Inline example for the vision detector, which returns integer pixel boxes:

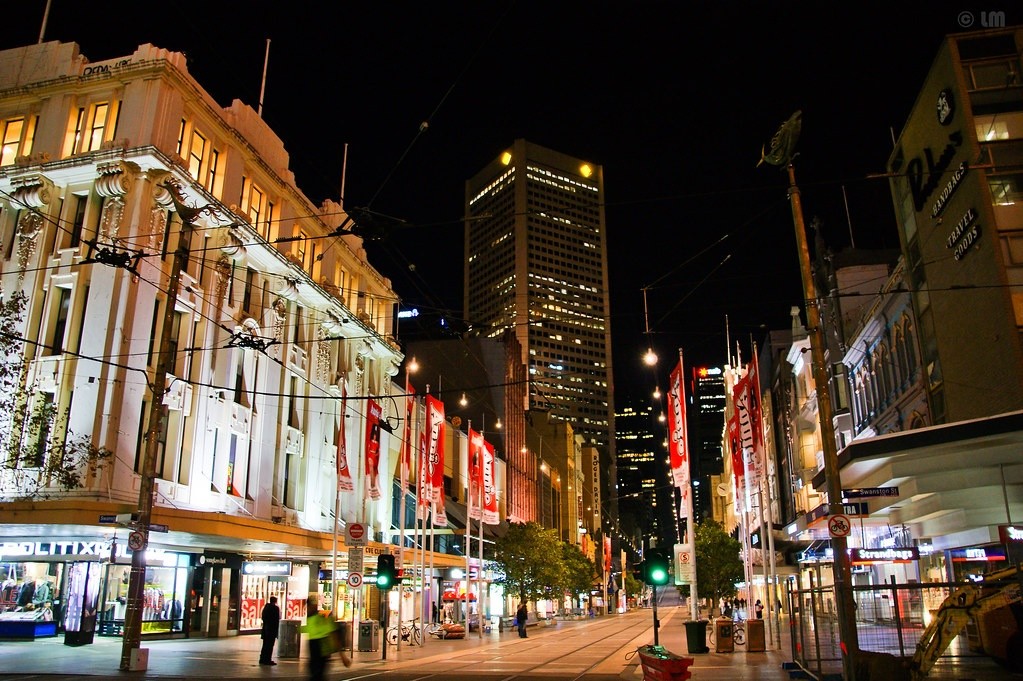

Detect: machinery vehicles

[852,562,1023,681]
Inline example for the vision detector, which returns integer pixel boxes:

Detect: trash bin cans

[713,616,734,652]
[683,621,711,653]
[358,620,379,653]
[278,619,301,659]
[334,620,352,652]
[744,619,766,651]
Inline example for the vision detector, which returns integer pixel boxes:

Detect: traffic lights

[646,547,669,586]
[376,554,395,590]
[632,559,647,582]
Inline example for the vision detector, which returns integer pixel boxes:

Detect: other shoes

[259,660,277,665]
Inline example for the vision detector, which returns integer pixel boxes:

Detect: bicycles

[386,616,425,645]
[709,617,745,645]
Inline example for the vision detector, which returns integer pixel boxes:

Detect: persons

[1,576,48,608]
[719,597,782,620]
[431,601,437,626]
[520,600,528,638]
[297,598,351,681]
[438,605,446,624]
[517,604,522,636]
[141,588,180,631]
[258,596,279,665]
